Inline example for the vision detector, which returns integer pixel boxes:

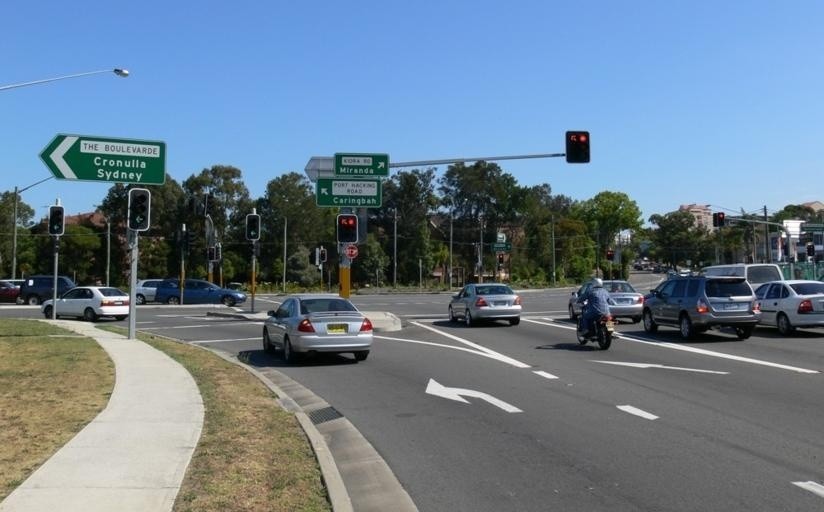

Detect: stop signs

[346,245,358,258]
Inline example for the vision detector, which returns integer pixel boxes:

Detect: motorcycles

[576,299,614,350]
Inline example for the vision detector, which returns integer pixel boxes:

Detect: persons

[578,278,610,336]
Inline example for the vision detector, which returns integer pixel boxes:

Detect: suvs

[643,275,762,338]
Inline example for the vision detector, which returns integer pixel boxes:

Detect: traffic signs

[491,243,511,252]
[334,153,389,177]
[315,178,382,208]
[38,133,165,185]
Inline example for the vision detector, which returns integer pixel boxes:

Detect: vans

[699,264,785,293]
[20,276,76,305]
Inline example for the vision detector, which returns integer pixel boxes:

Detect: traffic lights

[209,244,221,262]
[127,187,151,232]
[713,213,724,227]
[47,206,65,237]
[246,214,260,241]
[499,254,502,263]
[337,214,358,244]
[807,245,814,256]
[566,131,590,163]
[320,249,327,262]
[783,245,788,256]
[176,223,193,247]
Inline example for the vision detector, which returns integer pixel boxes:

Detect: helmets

[591,278,604,288]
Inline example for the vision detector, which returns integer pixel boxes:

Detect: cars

[755,279,824,336]
[136,279,247,306]
[568,280,644,323]
[633,262,692,278]
[40,286,130,323]
[262,295,373,364]
[0,281,24,305]
[448,284,522,327]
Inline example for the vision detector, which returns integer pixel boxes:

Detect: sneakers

[580,329,590,336]
